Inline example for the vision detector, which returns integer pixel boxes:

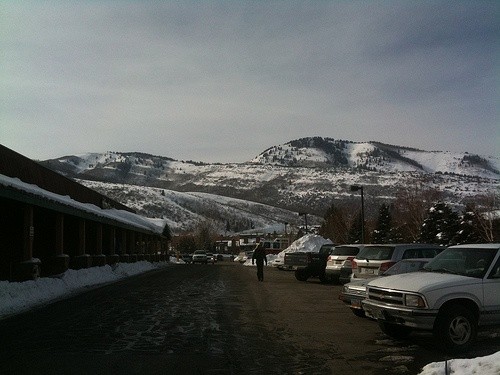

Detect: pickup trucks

[284,244,342,283]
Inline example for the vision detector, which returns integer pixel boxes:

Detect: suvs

[192,249,207,265]
[355,242,475,284]
[326,243,367,277]
[206,253,215,264]
[361,243,500,355]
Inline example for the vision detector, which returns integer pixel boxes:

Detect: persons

[251,242,268,282]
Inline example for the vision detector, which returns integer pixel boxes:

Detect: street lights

[351,184,365,244]
[300,210,309,236]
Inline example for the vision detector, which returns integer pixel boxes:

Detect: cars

[214,255,218,262]
[339,258,436,320]
[272,258,298,271]
